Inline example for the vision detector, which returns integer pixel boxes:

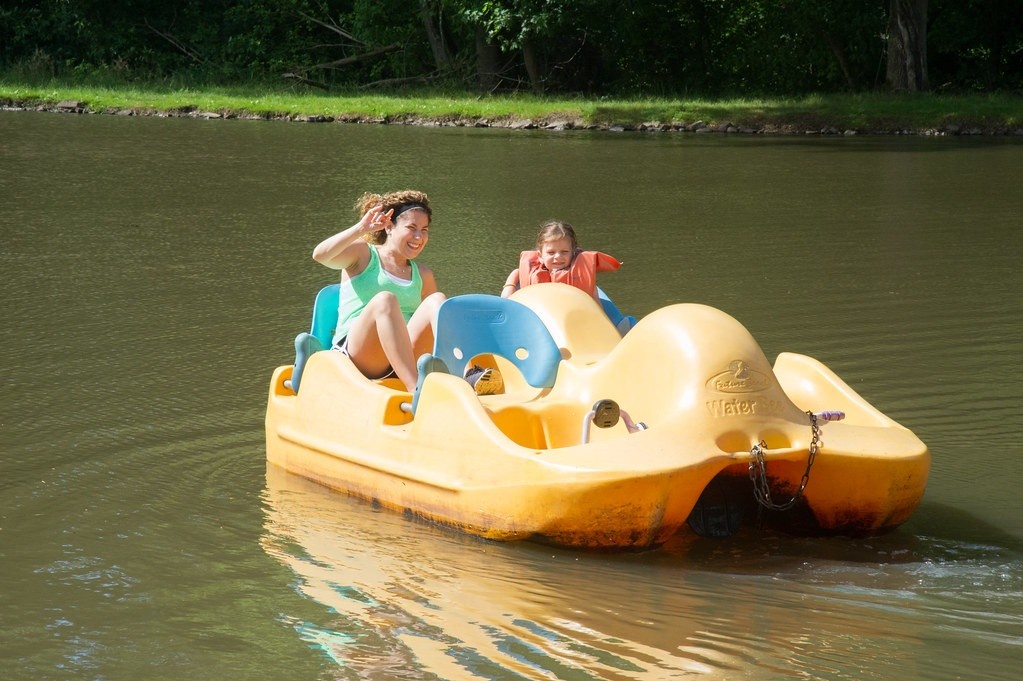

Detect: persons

[500,220,638,337]
[312,190,450,392]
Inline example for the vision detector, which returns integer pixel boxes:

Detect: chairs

[410,293,561,439]
[293,283,407,396]
[514,281,635,338]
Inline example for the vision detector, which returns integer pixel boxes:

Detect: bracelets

[502,284,517,288]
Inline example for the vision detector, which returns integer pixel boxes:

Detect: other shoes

[464,364,503,395]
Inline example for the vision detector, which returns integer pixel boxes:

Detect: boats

[264,283,933,549]
